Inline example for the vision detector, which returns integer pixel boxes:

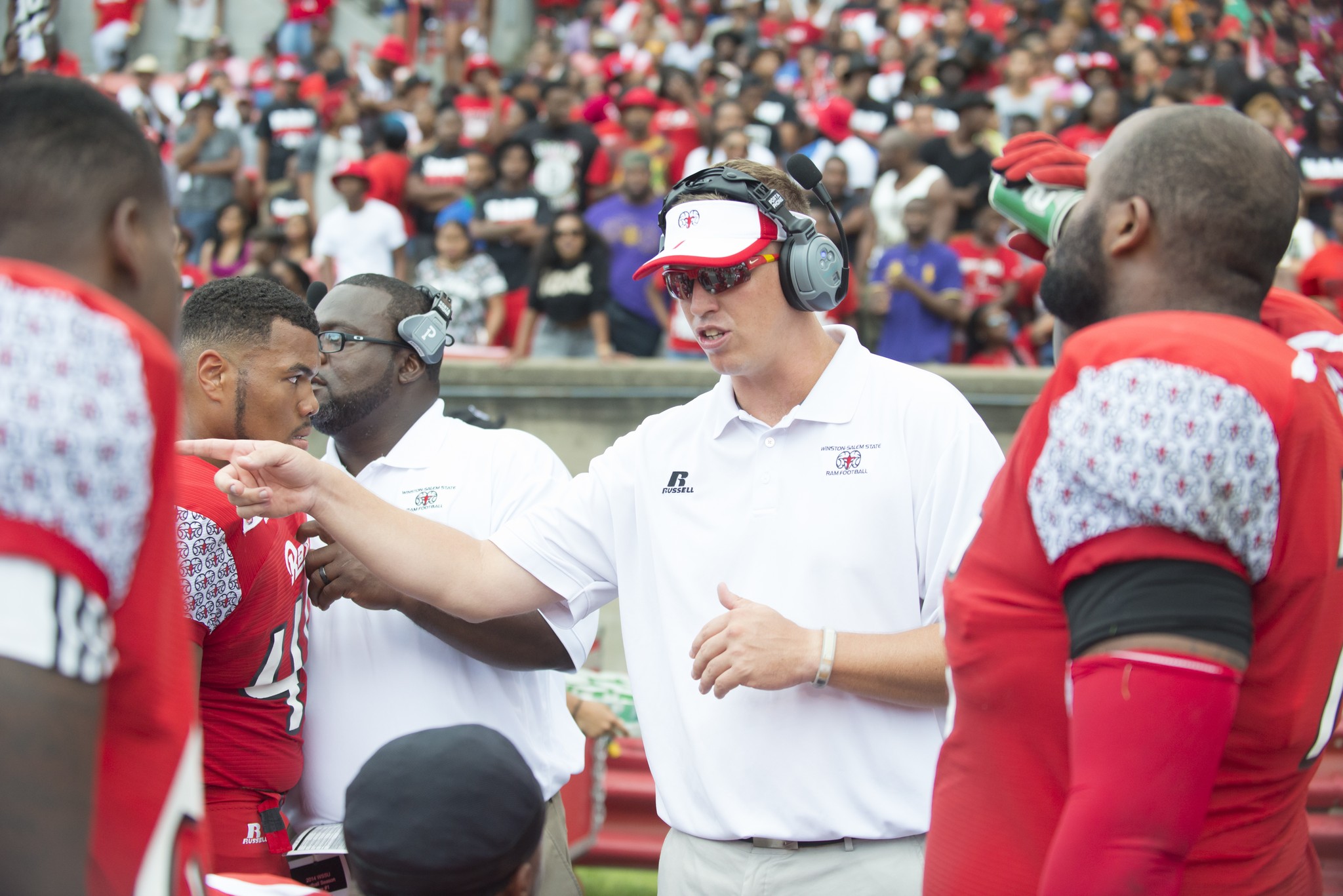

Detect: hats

[341,724,549,896]
[632,198,820,285]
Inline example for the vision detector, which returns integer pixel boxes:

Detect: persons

[285,272,600,895]
[0,0,1342,372]
[173,270,323,896]
[2,69,201,896]
[922,100,1343,896]
[340,722,553,896]
[562,690,631,743]
[171,160,1008,895]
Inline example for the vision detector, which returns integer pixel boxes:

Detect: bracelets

[571,695,584,722]
[811,627,838,690]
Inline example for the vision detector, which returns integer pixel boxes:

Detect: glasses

[661,254,779,299]
[317,330,416,354]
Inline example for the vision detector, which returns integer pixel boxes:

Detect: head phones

[397,284,453,364]
[657,167,848,311]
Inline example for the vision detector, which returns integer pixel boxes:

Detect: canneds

[987,166,1091,249]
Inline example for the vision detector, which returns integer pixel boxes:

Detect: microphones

[786,154,848,302]
[306,280,328,309]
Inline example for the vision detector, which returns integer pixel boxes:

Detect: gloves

[991,130,1093,264]
